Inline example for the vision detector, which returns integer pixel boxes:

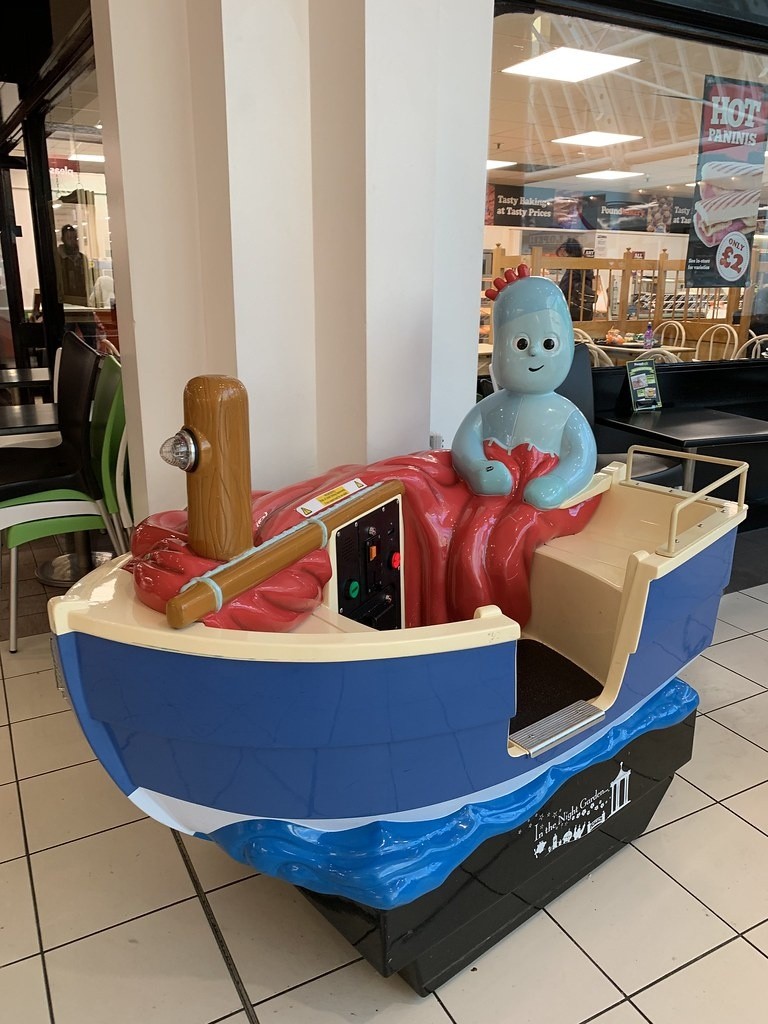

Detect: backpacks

[570,282,597,321]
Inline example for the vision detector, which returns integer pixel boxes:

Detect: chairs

[554,343,684,491]
[0,330,133,653]
[692,324,768,362]
[653,321,686,362]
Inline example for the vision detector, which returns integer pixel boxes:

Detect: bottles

[644,322,653,349]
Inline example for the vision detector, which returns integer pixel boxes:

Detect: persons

[55,225,98,348]
[551,237,596,323]
[751,273,768,356]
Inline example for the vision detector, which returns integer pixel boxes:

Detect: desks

[0,403,63,448]
[0,367,51,382]
[595,405,768,491]
[598,343,696,353]
[478,343,494,371]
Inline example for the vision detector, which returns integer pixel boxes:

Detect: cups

[608,329,619,343]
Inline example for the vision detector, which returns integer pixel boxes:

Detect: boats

[46,373,754,911]
